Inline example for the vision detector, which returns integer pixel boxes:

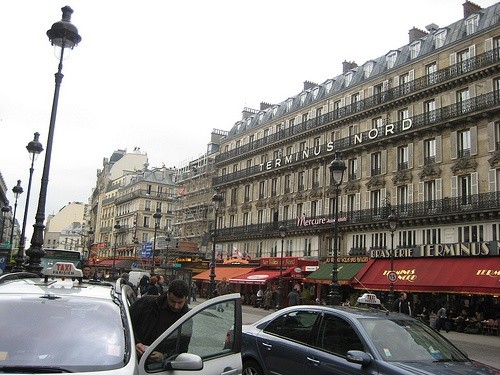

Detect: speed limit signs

[388,272,398,282]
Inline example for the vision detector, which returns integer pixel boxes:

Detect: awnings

[193,266,260,283]
[354,259,500,295]
[303,261,367,285]
[228,269,291,284]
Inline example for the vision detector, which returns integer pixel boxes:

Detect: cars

[224,292,500,375]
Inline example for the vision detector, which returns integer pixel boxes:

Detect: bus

[39,247,82,276]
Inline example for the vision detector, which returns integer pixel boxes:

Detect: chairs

[465,318,500,336]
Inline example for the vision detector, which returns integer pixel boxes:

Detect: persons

[300,287,309,305]
[257,287,262,308]
[214,276,228,312]
[436,303,451,333]
[475,311,482,320]
[127,278,194,364]
[191,281,197,304]
[263,287,272,309]
[309,283,317,299]
[287,287,300,308]
[139,273,165,298]
[395,293,411,312]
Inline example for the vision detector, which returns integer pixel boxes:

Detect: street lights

[386,209,397,310]
[112,220,120,279]
[25,5,83,275]
[13,132,46,273]
[89,229,93,258]
[0,201,10,242]
[163,235,171,275]
[275,221,288,311]
[150,208,162,277]
[328,150,347,305]
[10,179,24,246]
[207,186,223,299]
[133,240,139,265]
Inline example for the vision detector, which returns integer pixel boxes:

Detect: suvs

[0,262,243,375]
